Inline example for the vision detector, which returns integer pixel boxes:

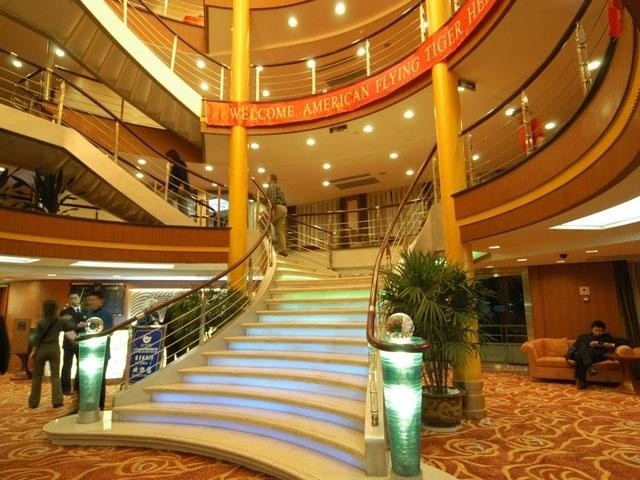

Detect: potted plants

[374,246,498,428]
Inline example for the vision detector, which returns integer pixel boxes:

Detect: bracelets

[611,343,615,349]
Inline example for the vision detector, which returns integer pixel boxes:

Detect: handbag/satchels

[27,345,39,369]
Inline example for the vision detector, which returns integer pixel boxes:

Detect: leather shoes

[589,368,598,376]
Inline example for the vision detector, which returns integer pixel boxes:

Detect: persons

[158,149,191,217]
[77,289,113,412]
[565,320,631,389]
[256,174,288,256]
[136,310,163,326]
[28,299,77,408]
[60,292,88,395]
[511,102,547,158]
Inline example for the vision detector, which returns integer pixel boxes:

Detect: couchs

[520,336,633,385]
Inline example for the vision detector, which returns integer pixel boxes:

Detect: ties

[77,309,80,319]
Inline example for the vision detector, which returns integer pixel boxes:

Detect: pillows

[542,337,568,356]
